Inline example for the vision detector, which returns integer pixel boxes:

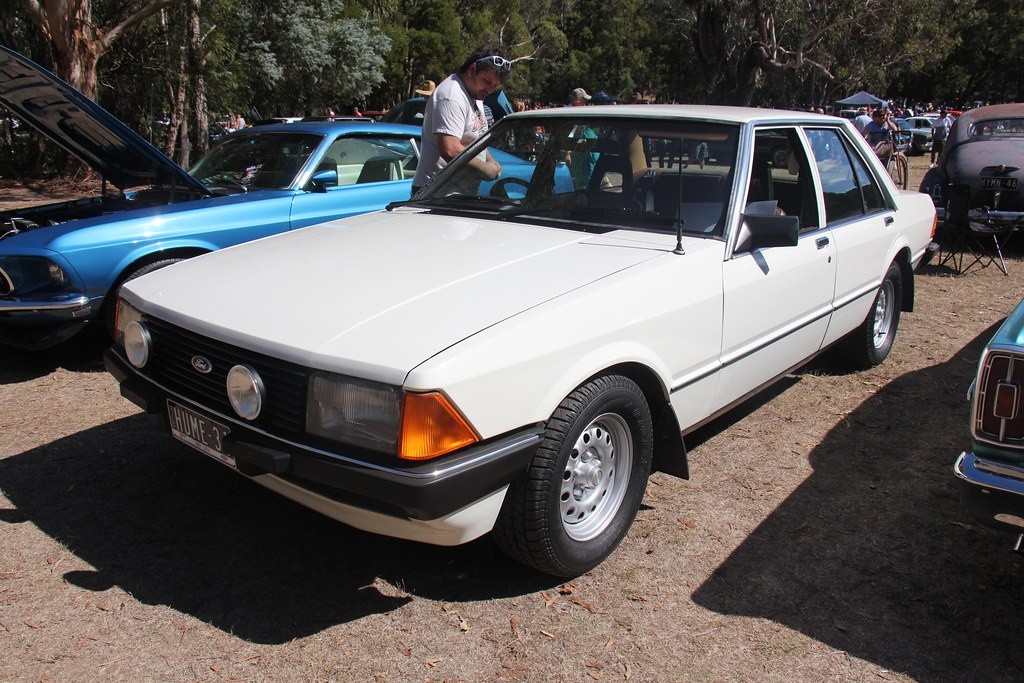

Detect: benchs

[629,172,823,223]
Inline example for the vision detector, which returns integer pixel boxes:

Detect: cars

[0,47,576,355]
[104,104,939,576]
[938,103,1024,232]
[215,89,991,158]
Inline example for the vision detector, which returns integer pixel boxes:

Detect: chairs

[282,155,337,170]
[936,185,1024,278]
[355,157,405,183]
[576,157,644,215]
[706,157,775,227]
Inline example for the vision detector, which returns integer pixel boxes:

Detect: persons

[894,97,946,110]
[411,44,511,200]
[227,113,235,129]
[930,110,952,167]
[414,80,435,100]
[861,110,898,156]
[656,139,708,169]
[548,88,592,166]
[236,114,245,130]
[816,108,823,114]
[352,107,362,118]
[514,99,564,112]
[995,100,999,104]
[853,108,873,133]
[324,107,335,116]
[806,104,814,113]
[986,100,990,105]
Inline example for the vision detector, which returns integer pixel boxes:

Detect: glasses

[475,56,511,72]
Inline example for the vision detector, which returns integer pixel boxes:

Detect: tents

[835,91,888,108]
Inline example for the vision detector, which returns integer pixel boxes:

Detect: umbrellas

[592,90,614,102]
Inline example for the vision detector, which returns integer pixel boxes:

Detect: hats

[415,80,435,95]
[573,88,592,100]
